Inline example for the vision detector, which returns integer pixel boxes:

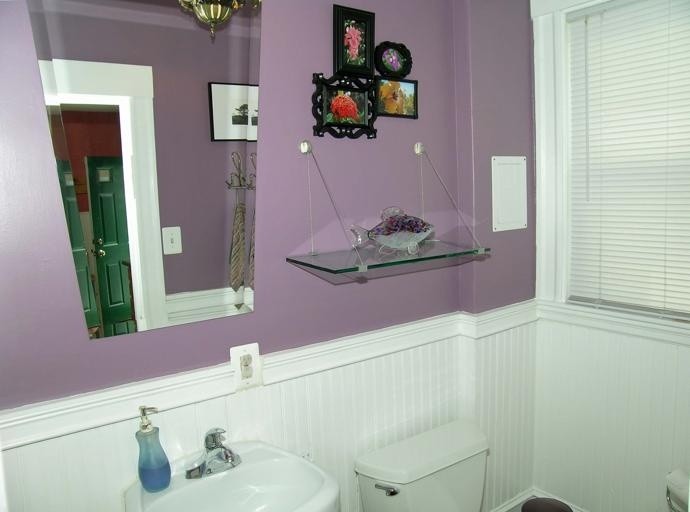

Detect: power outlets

[229,342,262,387]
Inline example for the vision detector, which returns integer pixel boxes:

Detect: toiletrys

[134,406,171,494]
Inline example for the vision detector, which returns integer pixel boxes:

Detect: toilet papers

[664,469,690,511]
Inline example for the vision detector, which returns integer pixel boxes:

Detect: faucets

[186,428,241,479]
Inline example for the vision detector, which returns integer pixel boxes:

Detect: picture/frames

[208,81,258,143]
[333,3,375,77]
[373,41,413,80]
[311,70,377,139]
[378,76,418,120]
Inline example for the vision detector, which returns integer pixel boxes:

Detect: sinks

[123,439,339,512]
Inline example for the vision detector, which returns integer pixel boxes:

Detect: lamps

[180,0,244,38]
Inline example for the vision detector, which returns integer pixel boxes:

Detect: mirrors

[24,1,260,340]
